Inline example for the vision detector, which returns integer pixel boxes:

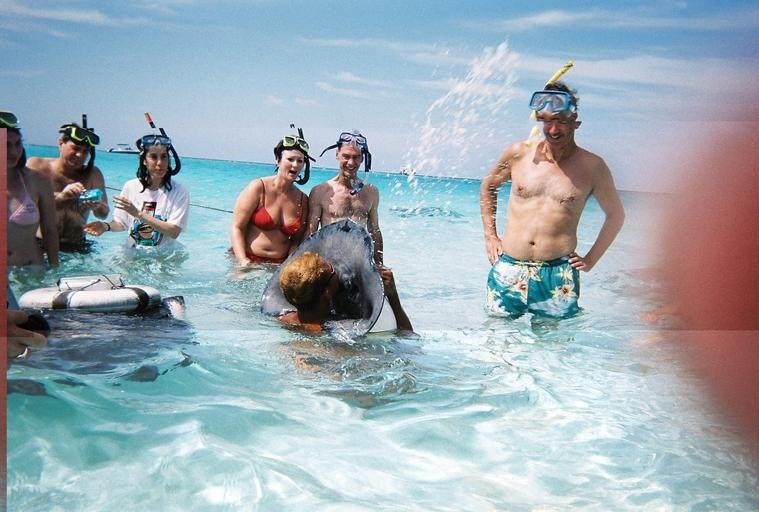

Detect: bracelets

[102,221,111,234]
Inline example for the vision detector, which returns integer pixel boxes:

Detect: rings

[16,346,29,359]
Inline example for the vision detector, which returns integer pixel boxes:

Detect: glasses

[527,91,572,114]
[0,110,19,129]
[328,262,335,283]
[140,134,172,146]
[66,123,100,148]
[338,131,367,147]
[283,136,310,156]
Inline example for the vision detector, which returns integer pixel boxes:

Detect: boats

[106,144,141,154]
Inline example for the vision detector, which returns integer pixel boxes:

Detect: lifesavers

[18,275,161,313]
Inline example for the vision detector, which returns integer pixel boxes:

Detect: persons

[300,128,386,272]
[272,252,414,338]
[25,112,110,257]
[0,109,61,275]
[5,302,46,371]
[82,124,190,251]
[478,83,629,325]
[225,133,316,269]
